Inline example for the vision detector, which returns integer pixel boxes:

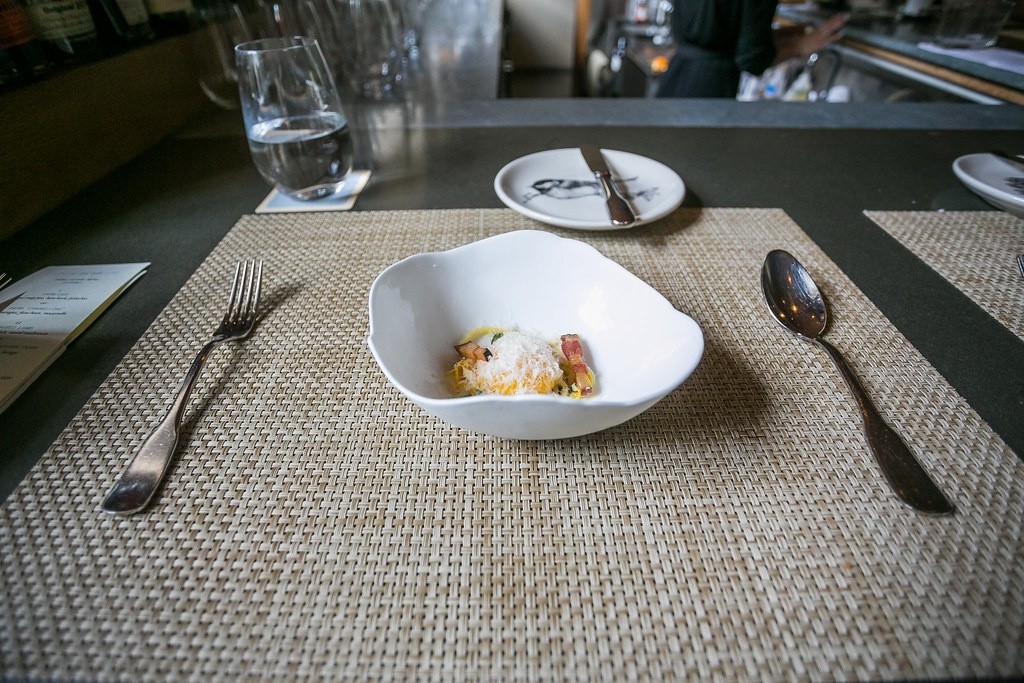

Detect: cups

[930,1,1018,47]
[235,0,419,200]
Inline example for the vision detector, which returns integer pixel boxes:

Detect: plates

[951,152,1024,220]
[494,147,687,231]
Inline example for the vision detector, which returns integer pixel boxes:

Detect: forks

[101,259,262,515]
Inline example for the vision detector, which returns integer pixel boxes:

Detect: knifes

[992,151,1024,166]
[580,142,635,226]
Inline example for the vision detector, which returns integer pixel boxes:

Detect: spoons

[761,249,954,517]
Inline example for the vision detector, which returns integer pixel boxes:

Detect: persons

[655,0,850,99]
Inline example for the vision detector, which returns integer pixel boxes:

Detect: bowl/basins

[367,229,706,441]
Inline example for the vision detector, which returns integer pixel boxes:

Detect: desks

[0,98,1024,683]
